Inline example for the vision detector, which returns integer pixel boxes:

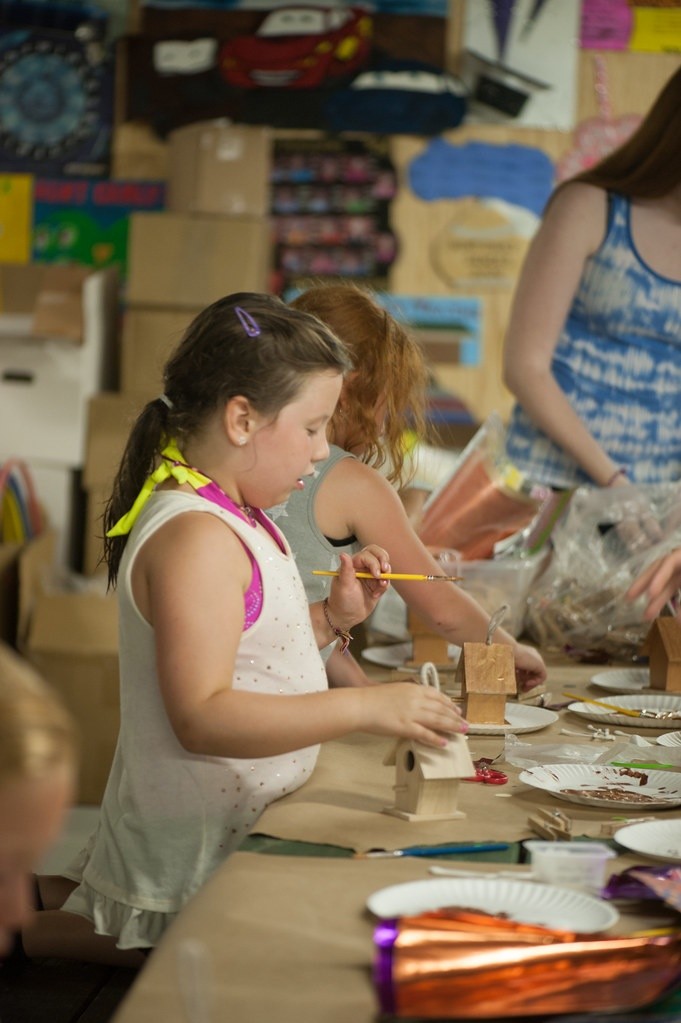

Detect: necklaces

[162,455,251,515]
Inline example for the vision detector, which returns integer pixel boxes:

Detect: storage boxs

[0,116,564,973]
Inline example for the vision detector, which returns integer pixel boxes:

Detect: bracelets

[323,596,354,655]
[608,468,627,490]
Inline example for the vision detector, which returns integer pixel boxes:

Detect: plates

[589,669,681,695]
[613,818,681,864]
[466,702,559,734]
[365,878,620,934]
[656,730,681,747]
[518,765,681,809]
[361,640,462,671]
[567,694,681,728]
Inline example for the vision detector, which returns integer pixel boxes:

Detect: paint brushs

[312,570,464,582]
[610,761,676,769]
[562,692,651,719]
[352,845,510,859]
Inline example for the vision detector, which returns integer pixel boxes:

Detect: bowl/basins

[371,541,550,638]
[522,840,617,888]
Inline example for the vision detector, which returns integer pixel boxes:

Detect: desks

[109,648,681,1023]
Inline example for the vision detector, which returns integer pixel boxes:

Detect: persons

[0,644,78,958]
[623,548,681,622]
[65,290,470,963]
[414,63,681,559]
[261,281,548,703]
